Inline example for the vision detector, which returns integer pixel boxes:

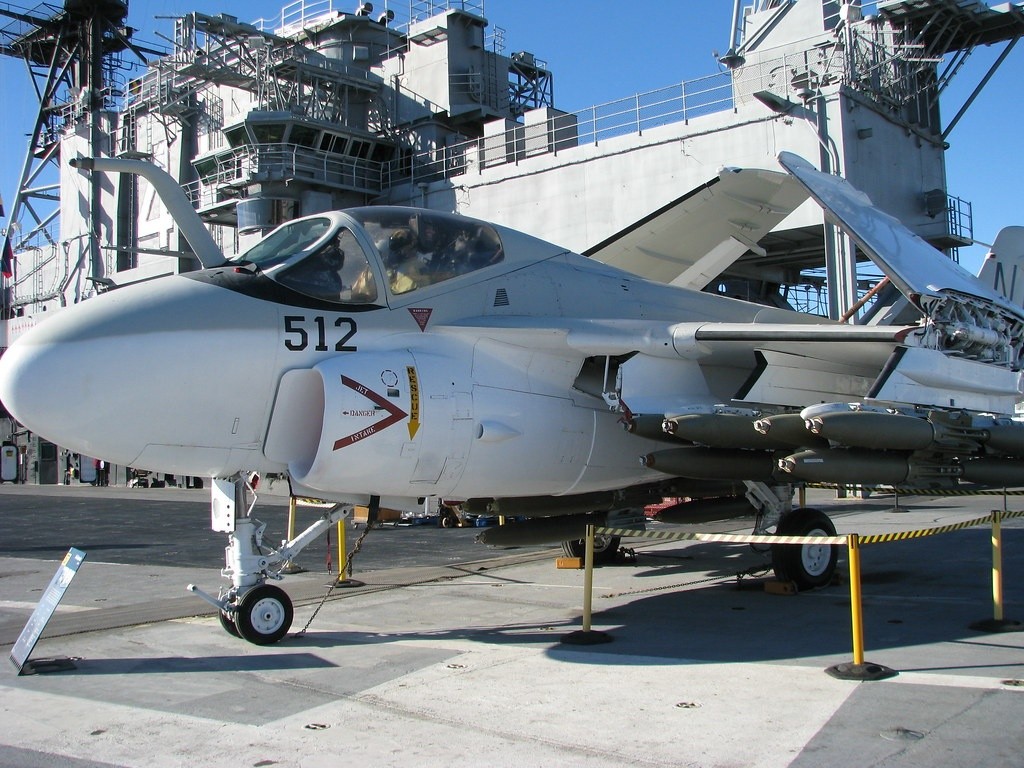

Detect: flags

[129,78,142,97]
[2,236,15,279]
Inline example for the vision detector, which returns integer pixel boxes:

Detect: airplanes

[1,149,1024,648]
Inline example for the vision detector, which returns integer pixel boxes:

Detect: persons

[127,468,139,489]
[68,464,74,480]
[350,229,430,303]
[93,458,101,486]
